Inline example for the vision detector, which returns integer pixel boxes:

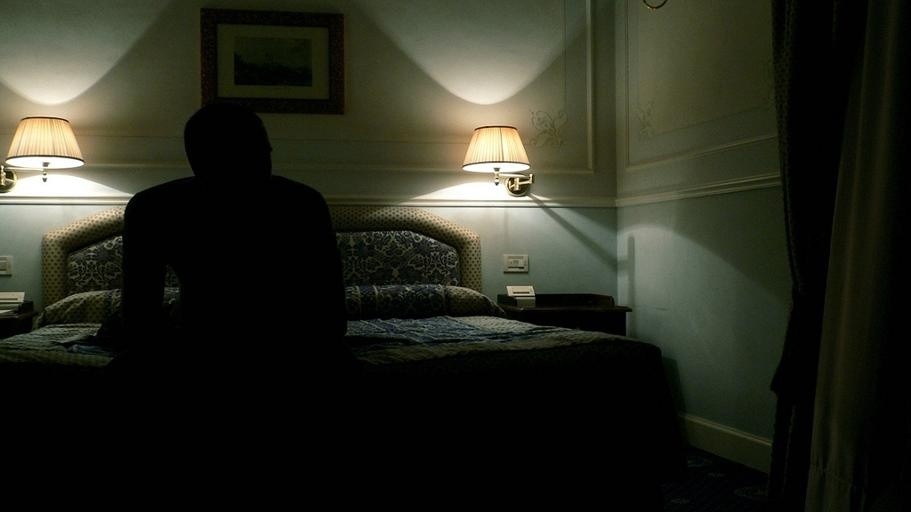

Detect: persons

[92,97,349,373]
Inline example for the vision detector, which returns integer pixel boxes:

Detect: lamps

[0,116,86,194]
[461,125,535,197]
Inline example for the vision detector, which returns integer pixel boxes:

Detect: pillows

[30,287,182,330]
[344,283,507,320]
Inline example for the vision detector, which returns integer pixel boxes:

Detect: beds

[1,205,689,512]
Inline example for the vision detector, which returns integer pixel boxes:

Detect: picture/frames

[199,8,345,115]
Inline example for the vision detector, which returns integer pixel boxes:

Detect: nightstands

[496,293,633,337]
[0,292,42,342]
